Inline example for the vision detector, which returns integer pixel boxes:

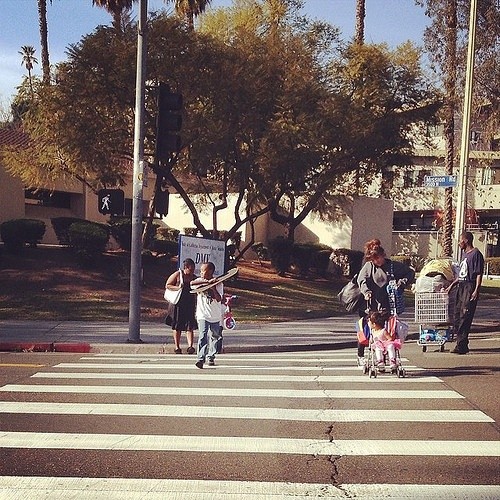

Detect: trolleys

[413,282,460,353]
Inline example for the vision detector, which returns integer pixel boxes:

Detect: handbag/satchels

[163,288,182,305]
[337,281,364,314]
[387,284,406,315]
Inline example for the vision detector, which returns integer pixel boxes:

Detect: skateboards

[189,267,239,296]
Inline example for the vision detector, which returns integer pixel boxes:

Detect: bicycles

[220,294,238,331]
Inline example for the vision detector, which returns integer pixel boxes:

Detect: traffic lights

[154,81,183,155]
[98,188,125,215]
[155,189,170,217]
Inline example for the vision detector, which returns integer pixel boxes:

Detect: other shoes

[174,348,182,354]
[357,357,366,367]
[451,344,469,354]
[209,360,214,365]
[187,347,195,354]
[378,361,385,372]
[195,362,203,369]
[391,364,397,374]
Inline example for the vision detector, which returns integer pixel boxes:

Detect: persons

[190,262,223,368]
[445,231,485,354]
[166,258,197,354]
[217,297,227,354]
[357,239,381,366]
[371,312,397,369]
[357,238,415,366]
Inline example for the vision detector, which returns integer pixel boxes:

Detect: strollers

[362,293,406,379]
